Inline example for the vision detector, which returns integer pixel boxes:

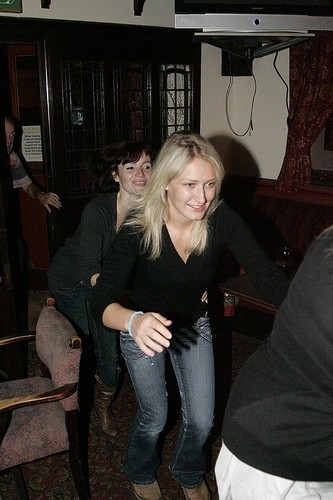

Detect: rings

[54,196,56,199]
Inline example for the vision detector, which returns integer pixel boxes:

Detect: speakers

[221,50,253,77]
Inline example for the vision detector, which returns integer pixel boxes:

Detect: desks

[218,278,276,398]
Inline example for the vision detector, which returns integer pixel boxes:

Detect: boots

[93,375,119,436]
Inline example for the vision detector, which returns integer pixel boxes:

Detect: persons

[45,140,209,439]
[214,224,333,500]
[90,131,293,500]
[4,117,62,324]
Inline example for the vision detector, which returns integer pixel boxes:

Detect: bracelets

[128,311,144,337]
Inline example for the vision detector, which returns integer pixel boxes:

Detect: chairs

[0,298,95,500]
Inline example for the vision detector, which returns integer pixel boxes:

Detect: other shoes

[181,479,211,500]
[131,479,163,500]
